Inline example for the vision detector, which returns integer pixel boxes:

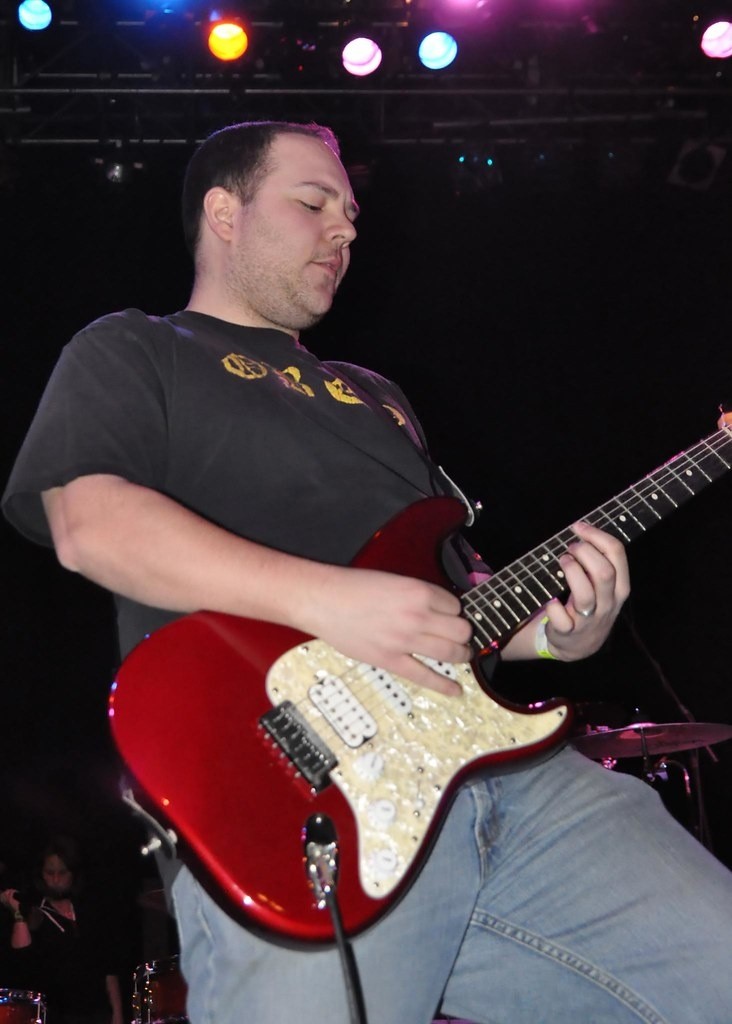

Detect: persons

[0,124,731,1024]
[629,746,694,829]
[0,833,126,1022]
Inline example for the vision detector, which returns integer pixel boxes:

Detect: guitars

[111,407,732,939]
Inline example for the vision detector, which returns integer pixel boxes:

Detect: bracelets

[8,910,25,925]
[532,604,561,662]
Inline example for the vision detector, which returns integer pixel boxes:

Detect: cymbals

[578,716,731,763]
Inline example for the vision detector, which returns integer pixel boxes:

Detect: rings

[571,597,599,618]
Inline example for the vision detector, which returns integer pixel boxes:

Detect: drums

[1,953,188,1024]
